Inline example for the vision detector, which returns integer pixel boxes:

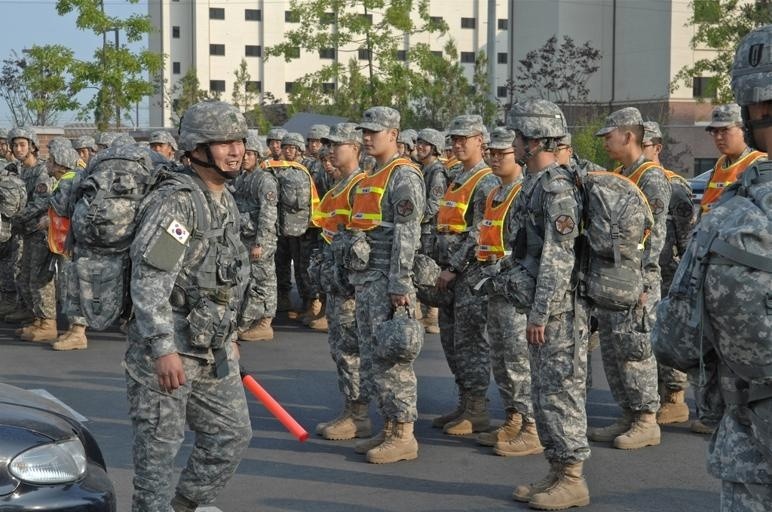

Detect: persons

[309,122,377,441]
[587,106,672,450]
[148,131,177,162]
[416,128,448,336]
[72,136,97,162]
[690,104,769,433]
[278,133,312,312]
[289,123,330,319]
[346,106,427,466]
[302,147,340,331]
[49,147,88,350]
[508,99,591,511]
[123,100,253,512]
[6,292,34,322]
[0,129,18,318]
[8,126,54,343]
[475,127,545,457]
[396,134,414,160]
[232,137,278,342]
[430,114,503,436]
[648,25,772,511]
[644,120,698,425]
[266,128,288,162]
[555,131,606,176]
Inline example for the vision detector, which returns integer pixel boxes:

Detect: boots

[323,402,375,439]
[367,423,419,464]
[417,308,440,335]
[691,420,717,434]
[415,301,422,319]
[15,317,41,337]
[614,411,660,450]
[432,392,467,428]
[296,299,322,322]
[48,324,73,346]
[591,408,633,442]
[239,318,274,342]
[315,398,352,435]
[21,319,57,341]
[53,324,87,351]
[478,410,523,446]
[443,395,491,435]
[0,291,19,320]
[657,390,689,424]
[303,302,326,326]
[512,458,560,502]
[5,300,36,324]
[354,417,396,454]
[308,315,329,333]
[528,460,590,510]
[493,418,546,457]
[277,294,293,311]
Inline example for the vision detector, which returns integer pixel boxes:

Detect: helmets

[178,103,248,154]
[95,132,117,148]
[245,135,263,158]
[49,146,79,170]
[8,128,40,152]
[47,137,72,149]
[306,125,329,141]
[0,129,10,139]
[280,133,306,154]
[732,24,772,106]
[112,134,135,147]
[397,132,414,152]
[73,135,98,151]
[403,130,417,144]
[417,128,445,155]
[482,125,491,145]
[266,129,288,146]
[506,99,567,139]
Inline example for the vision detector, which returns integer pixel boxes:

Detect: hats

[355,106,400,131]
[169,135,178,151]
[642,122,662,143]
[596,108,643,137]
[445,115,483,138]
[484,126,516,151]
[705,103,742,131]
[147,131,171,146]
[558,132,572,146]
[320,123,364,144]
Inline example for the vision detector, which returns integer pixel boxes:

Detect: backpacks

[269,163,311,237]
[67,143,169,331]
[573,163,654,312]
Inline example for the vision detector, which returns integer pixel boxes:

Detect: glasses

[555,147,567,155]
[451,135,479,146]
[710,126,736,136]
[489,152,514,160]
[283,146,297,149]
[327,143,352,147]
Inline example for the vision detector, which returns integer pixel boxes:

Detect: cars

[0,383,118,512]
[686,168,715,205]
